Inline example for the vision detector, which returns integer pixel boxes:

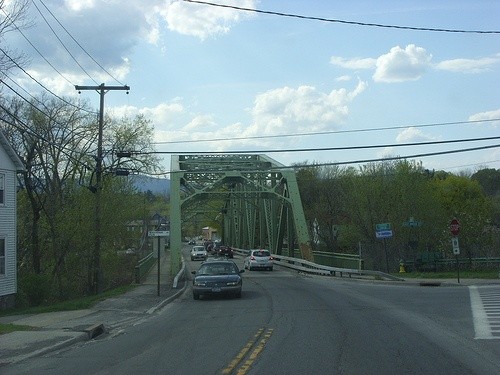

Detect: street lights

[153,219,168,296]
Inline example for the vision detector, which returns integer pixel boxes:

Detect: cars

[188,239,197,245]
[190,259,246,300]
[242,249,274,271]
[189,246,209,261]
[215,245,234,259]
[198,237,221,255]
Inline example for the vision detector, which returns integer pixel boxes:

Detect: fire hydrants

[399,258,406,273]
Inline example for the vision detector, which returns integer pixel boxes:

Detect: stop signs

[449,219,460,235]
